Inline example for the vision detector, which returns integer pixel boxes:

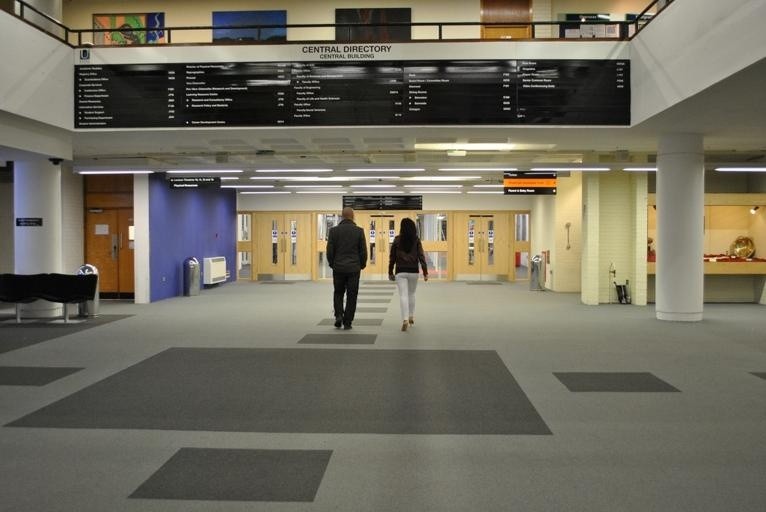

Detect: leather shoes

[334,316,343,328]
[343,324,352,330]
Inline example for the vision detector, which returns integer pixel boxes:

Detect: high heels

[400,320,409,331]
[409,319,415,327]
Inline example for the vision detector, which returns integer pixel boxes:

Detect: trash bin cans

[76,263,100,319]
[183,257,201,296]
[529,255,546,291]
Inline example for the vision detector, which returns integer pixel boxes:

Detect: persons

[388,217,428,331]
[324,207,367,330]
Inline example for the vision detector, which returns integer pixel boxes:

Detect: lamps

[750,206,761,214]
